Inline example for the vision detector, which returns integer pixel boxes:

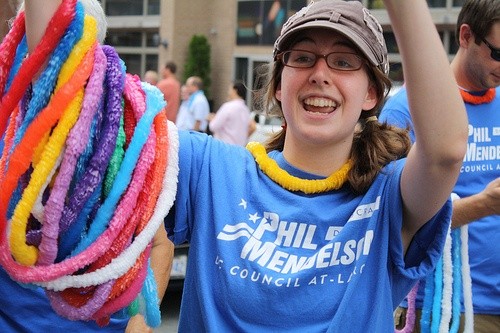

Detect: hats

[272,0,389,78]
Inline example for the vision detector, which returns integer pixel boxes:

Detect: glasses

[472,29,500,61]
[277,48,372,71]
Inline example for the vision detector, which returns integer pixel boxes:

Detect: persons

[143,63,255,146]
[0,0,174,333]
[26,0,469,333]
[379,0,500,333]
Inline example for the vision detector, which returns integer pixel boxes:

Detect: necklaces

[461,88,497,105]
[246,142,358,195]
[393,193,474,333]
[0,0,180,328]
